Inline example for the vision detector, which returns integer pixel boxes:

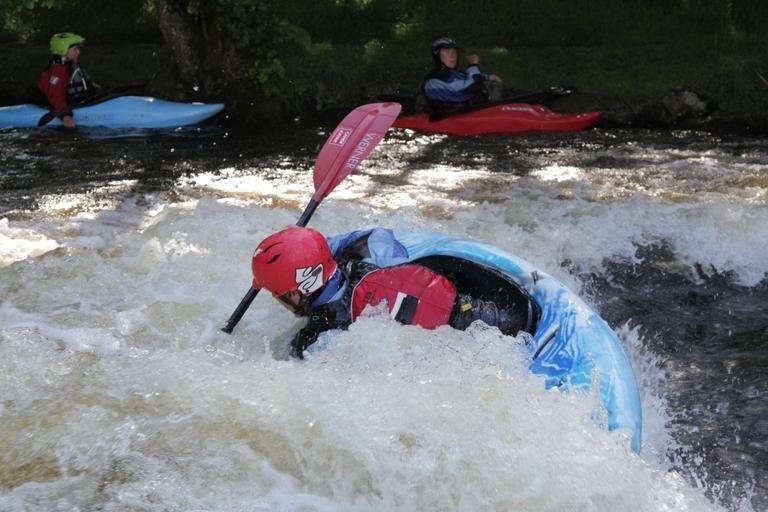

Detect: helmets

[49,32,85,55]
[249,225,336,301]
[430,37,460,60]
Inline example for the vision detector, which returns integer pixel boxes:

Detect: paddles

[429,87,556,122]
[38,91,97,129]
[222,100,401,334]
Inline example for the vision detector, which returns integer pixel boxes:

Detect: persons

[421,36,504,119]
[250,219,517,362]
[38,32,103,130]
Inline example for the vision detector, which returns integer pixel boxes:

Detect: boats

[0,93,228,130]
[335,229,644,473]
[389,103,601,138]
